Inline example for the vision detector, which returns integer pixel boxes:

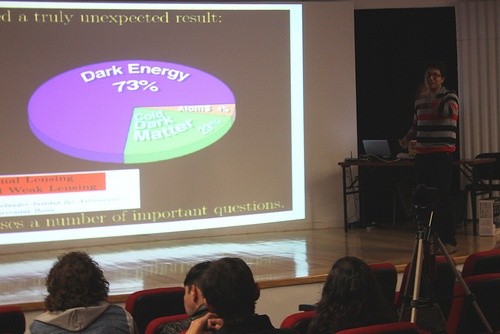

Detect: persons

[153,261,217,334]
[399,62,460,255]
[186,257,298,334]
[29,252,139,334]
[293,256,399,334]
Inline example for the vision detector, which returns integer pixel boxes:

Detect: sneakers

[437,243,457,254]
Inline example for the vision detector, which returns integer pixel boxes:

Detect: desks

[338,159,497,236]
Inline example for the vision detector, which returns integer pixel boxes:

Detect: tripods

[399,210,494,334]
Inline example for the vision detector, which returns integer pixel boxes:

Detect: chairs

[0,248,500,334]
[466,153,500,236]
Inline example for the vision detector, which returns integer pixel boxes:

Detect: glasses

[424,72,442,79]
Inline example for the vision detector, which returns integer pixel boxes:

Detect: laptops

[363,140,392,159]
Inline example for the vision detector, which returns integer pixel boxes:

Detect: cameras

[411,184,440,208]
